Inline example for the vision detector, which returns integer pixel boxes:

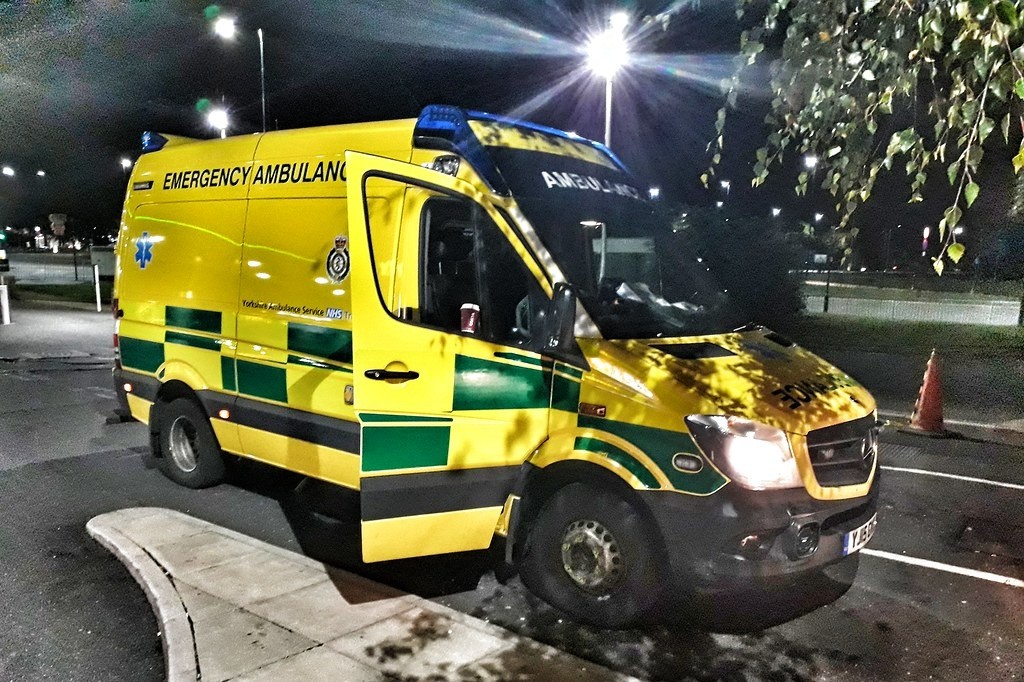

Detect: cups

[460,303,480,333]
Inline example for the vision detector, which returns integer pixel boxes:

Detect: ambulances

[112,105,889,627]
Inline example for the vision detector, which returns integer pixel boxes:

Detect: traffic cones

[895,348,950,440]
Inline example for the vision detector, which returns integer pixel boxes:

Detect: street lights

[592,28,625,149]
[215,17,266,133]
